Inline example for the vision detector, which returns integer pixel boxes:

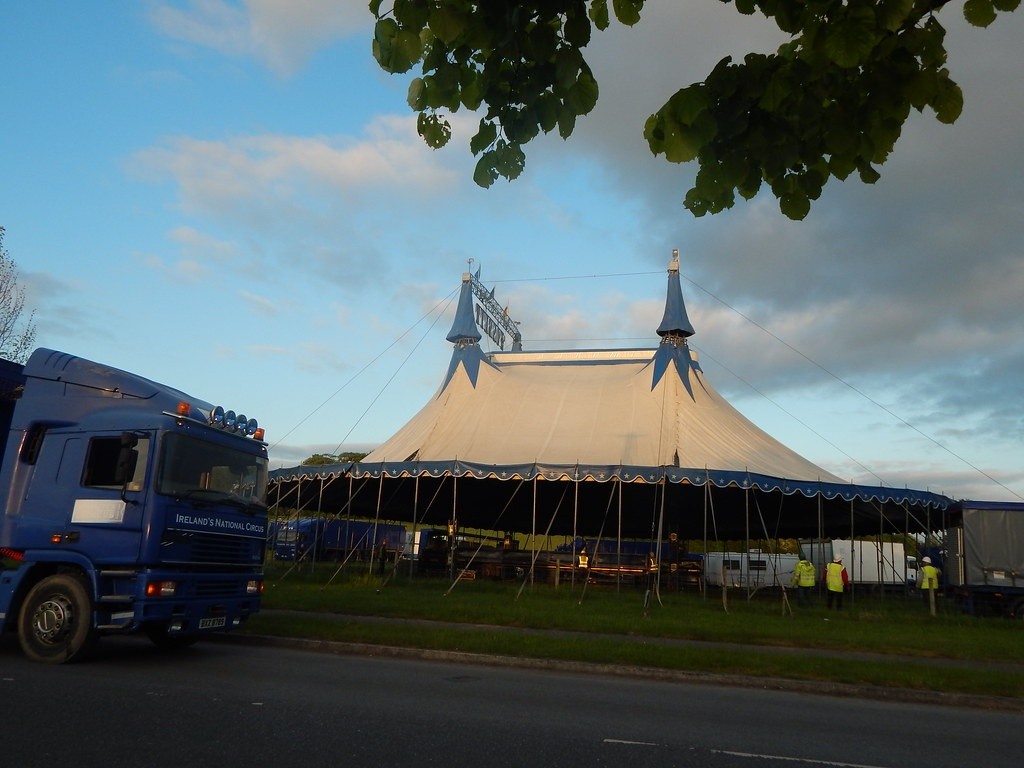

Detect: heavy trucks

[276,516,407,566]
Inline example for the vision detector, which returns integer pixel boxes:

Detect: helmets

[922,556,931,564]
[798,552,806,560]
[833,554,844,562]
[650,552,654,557]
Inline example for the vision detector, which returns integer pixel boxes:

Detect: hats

[581,551,586,554]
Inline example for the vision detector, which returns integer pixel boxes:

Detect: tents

[266,347,954,546]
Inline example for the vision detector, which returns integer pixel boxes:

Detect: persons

[789,551,816,609]
[576,550,590,583]
[645,552,658,587]
[823,554,848,611]
[379,541,387,567]
[916,557,941,609]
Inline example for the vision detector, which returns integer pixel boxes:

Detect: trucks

[947,499,1024,620]
[0,348,279,666]
[556,536,671,555]
[415,526,520,563]
[798,538,921,598]
[702,550,801,600]
